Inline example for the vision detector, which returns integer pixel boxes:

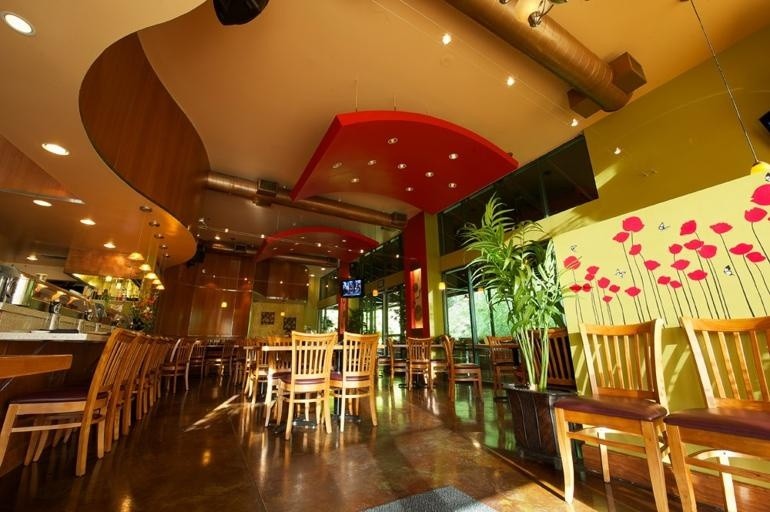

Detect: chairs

[206,326,378,442]
[488,324,573,398]
[385,335,482,400]
[552,314,770,512]
[0,325,211,478]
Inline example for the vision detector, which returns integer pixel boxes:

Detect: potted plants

[456,190,581,472]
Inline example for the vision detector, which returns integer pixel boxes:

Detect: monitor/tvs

[341,278,363,298]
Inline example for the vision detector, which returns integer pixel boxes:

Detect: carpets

[364,484,501,512]
